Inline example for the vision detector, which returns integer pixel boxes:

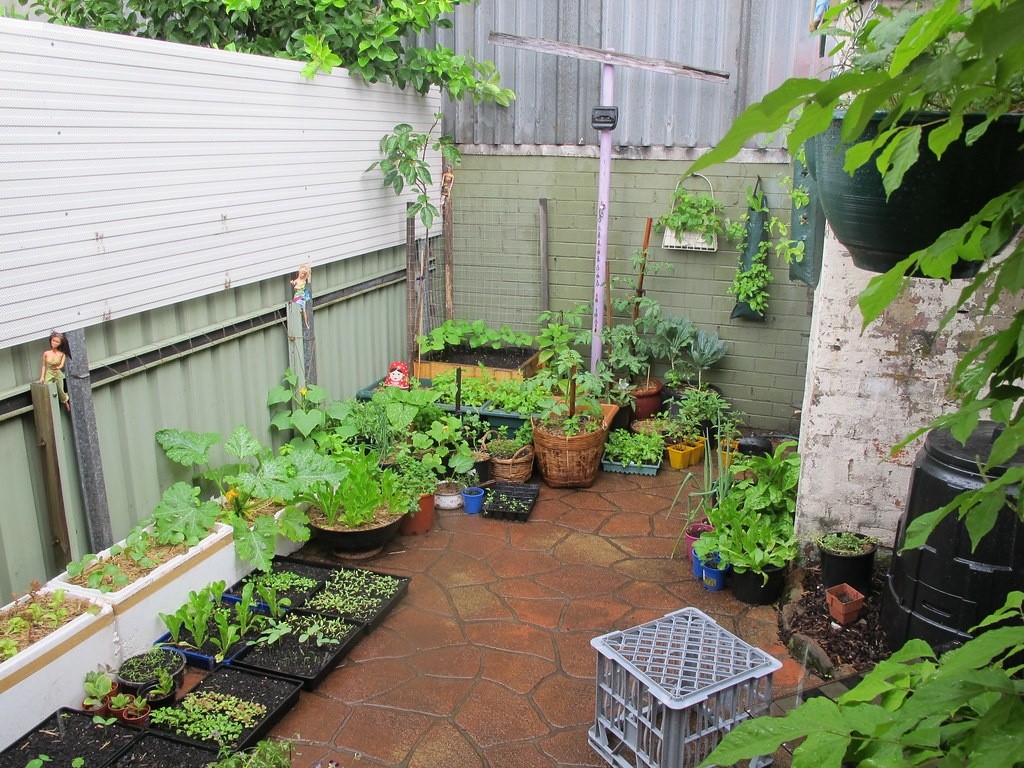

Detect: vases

[826,583,864,626]
[202,485,338,556]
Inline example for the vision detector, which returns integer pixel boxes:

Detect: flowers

[146,427,340,572]
[267,369,358,452]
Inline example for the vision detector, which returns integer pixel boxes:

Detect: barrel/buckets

[385,361,410,389]
[878,422,1023,649]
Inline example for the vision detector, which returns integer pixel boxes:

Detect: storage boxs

[585,608,782,768]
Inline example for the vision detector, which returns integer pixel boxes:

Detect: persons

[40,333,71,414]
[439,164,454,215]
[289,259,310,330]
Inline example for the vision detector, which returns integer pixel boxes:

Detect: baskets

[531,414,609,489]
[482,431,535,484]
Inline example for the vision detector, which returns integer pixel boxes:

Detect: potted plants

[357,113,497,450]
[819,532,876,597]
[632,396,687,460]
[687,416,706,446]
[651,316,728,449]
[556,349,619,452]
[478,421,535,484]
[481,377,556,442]
[414,319,542,394]
[53,513,237,666]
[666,428,801,606]
[684,0,1023,277]
[462,410,491,481]
[667,418,690,469]
[533,397,606,487]
[609,249,674,418]
[459,470,483,512]
[680,416,702,466]
[717,411,744,468]
[1,556,412,767]
[421,452,475,509]
[602,429,663,475]
